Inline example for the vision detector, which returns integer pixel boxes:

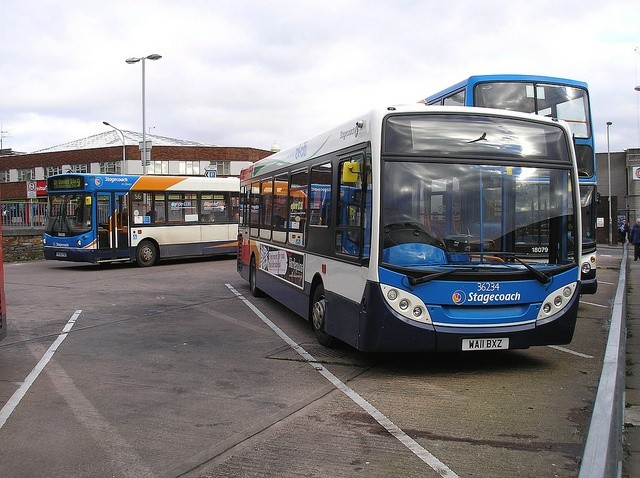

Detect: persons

[117,208,128,226]
[74,198,88,226]
[631,218,640,261]
[620,219,628,246]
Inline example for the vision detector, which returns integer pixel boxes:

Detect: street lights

[125,54,162,175]
[102,121,124,174]
[606,122,613,245]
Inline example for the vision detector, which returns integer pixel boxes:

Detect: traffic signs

[27,181,36,198]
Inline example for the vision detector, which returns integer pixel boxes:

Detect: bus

[236,106,581,354]
[43,171,239,267]
[303,184,372,259]
[417,74,601,294]
[241,181,307,232]
